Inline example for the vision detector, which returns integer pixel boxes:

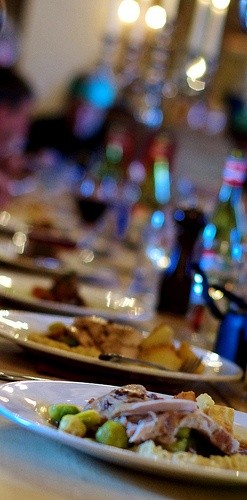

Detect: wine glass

[58,174,117,281]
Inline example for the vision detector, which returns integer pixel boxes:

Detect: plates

[0,269,152,320]
[0,380,247,489]
[0,198,139,280]
[0,310,242,390]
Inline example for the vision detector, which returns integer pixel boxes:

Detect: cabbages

[47,404,128,448]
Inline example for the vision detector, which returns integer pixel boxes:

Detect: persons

[0,60,247,228]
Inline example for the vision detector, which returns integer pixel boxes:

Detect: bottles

[123,130,176,246]
[189,146,247,333]
[155,207,207,314]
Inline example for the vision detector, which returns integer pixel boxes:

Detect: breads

[174,392,235,435]
